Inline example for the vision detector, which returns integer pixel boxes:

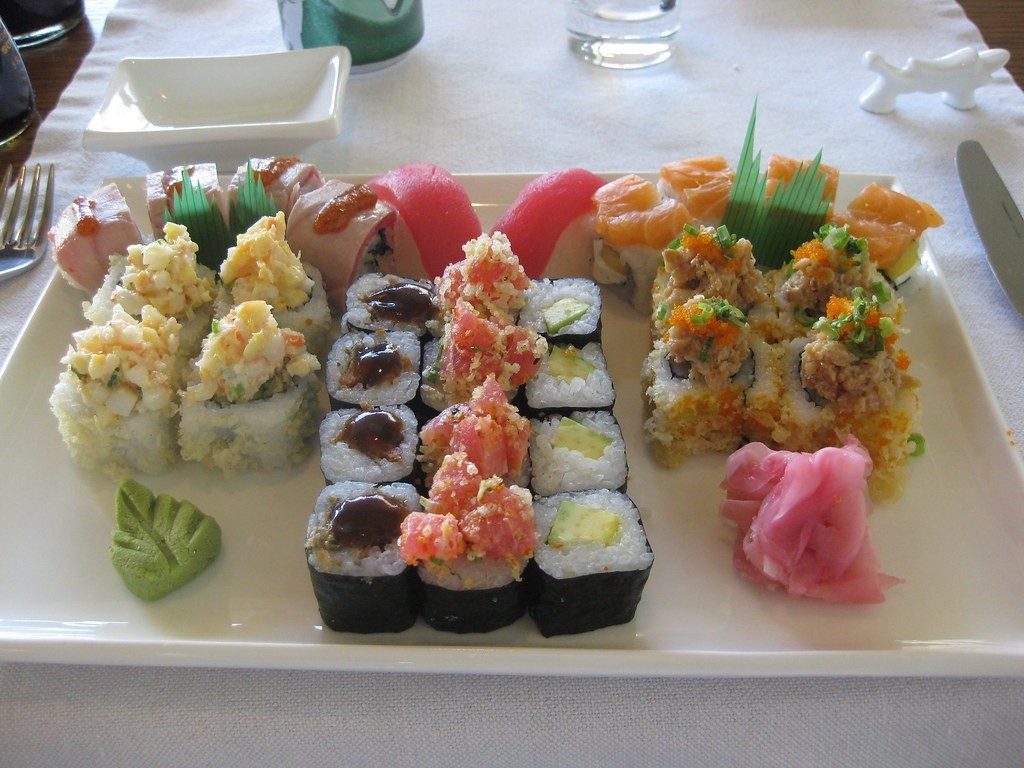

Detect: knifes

[956,139,1024,321]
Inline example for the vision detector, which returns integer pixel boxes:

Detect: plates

[0,169,1024,678]
[82,44,352,153]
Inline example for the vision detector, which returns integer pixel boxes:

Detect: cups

[562,1,683,69]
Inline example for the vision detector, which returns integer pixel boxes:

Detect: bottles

[0,0,86,146]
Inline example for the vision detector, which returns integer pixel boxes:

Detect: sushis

[47,156,946,637]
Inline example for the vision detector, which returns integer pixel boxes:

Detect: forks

[0,162,57,283]
